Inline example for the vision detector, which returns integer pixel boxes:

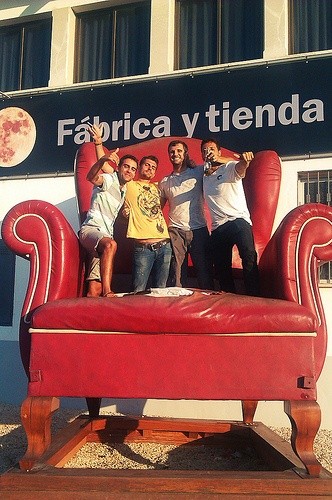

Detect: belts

[134,239,170,251]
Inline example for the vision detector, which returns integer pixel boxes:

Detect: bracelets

[94,140,103,145]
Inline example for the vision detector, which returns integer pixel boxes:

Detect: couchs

[0,134,332,499]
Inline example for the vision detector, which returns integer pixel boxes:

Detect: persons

[199,135,265,298]
[77,147,139,298]
[120,139,236,289]
[84,121,173,295]
